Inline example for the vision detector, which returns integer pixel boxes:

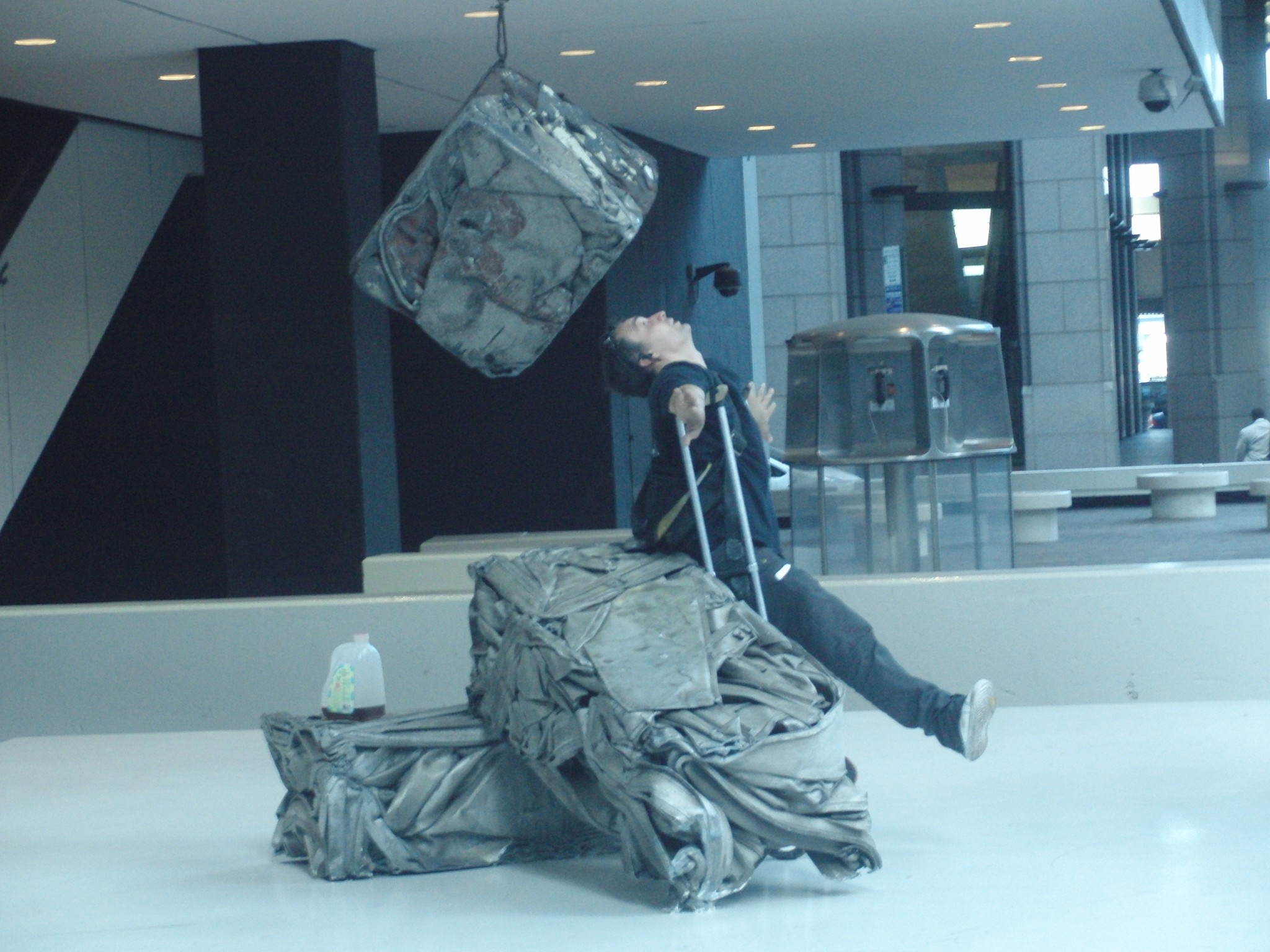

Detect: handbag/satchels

[630,462,725,552]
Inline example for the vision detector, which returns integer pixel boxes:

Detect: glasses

[599,333,624,360]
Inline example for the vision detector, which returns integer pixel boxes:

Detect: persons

[1234,407,1270,462]
[600,311,995,762]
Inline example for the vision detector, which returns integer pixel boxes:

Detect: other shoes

[958,679,997,761]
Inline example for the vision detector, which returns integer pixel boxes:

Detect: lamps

[686,262,742,304]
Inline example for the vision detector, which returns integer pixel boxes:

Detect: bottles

[320,635,386,723]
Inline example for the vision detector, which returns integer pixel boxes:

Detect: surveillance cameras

[712,267,741,297]
[1138,72,1176,113]
